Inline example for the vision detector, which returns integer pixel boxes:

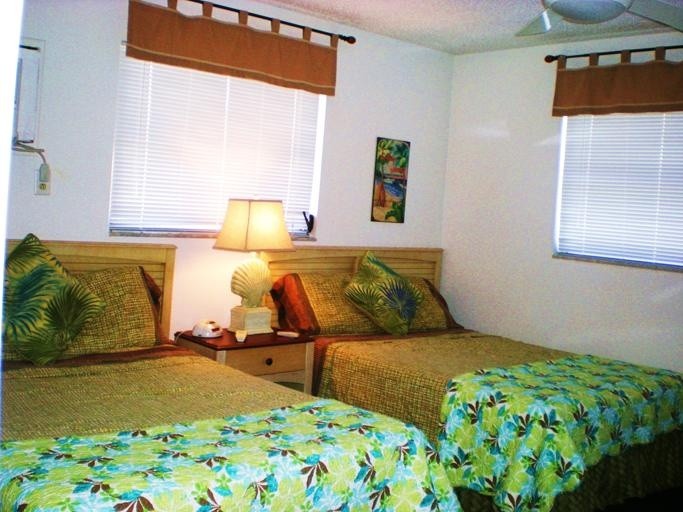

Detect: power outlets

[34,169,50,196]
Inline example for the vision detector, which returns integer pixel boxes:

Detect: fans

[513,0,683,37]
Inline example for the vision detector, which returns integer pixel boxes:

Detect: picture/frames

[371,137,411,223]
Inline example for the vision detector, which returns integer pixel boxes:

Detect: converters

[40,164,50,182]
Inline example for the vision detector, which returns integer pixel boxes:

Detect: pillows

[1,232,106,370]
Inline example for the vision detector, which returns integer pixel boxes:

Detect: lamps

[214,199,297,336]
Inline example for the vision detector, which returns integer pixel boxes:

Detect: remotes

[277,331,299,338]
